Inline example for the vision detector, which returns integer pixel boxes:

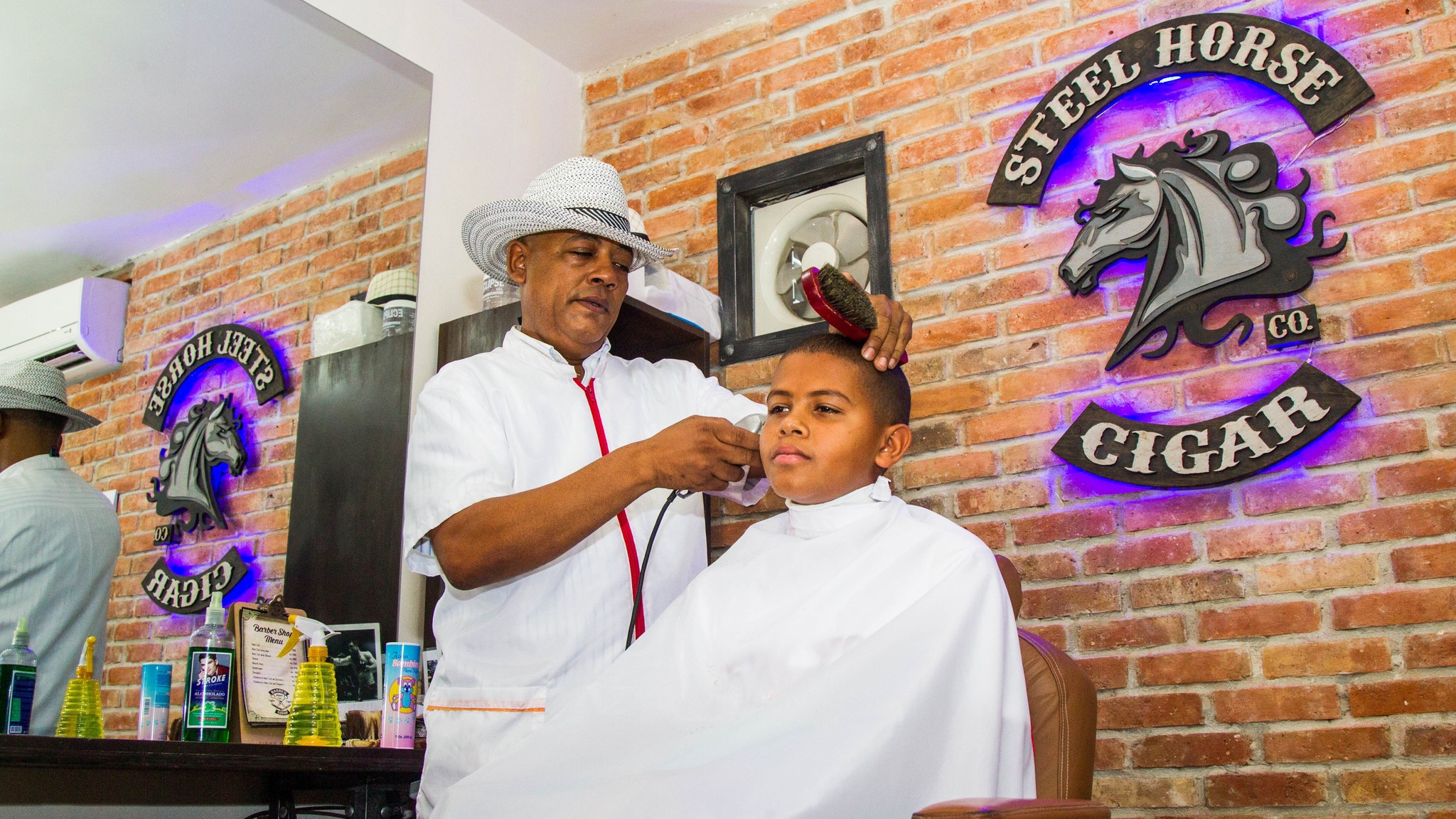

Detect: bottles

[181,590,236,742]
[382,299,418,339]
[137,662,172,740]
[380,642,421,749]
[0,618,38,735]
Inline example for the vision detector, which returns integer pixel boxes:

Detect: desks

[1,732,427,818]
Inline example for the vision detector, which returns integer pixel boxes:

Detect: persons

[404,157,913,819]
[1,360,121,739]
[424,331,1038,819]
[198,653,230,679]
[327,640,376,700]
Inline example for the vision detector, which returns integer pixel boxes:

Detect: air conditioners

[1,274,131,386]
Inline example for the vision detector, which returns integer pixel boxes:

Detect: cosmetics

[183,592,237,742]
[0,617,39,734]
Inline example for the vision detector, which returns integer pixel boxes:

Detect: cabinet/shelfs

[437,293,712,570]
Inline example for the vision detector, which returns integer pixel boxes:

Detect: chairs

[911,554,1113,819]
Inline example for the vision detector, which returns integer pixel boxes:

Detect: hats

[0,360,103,433]
[460,157,679,286]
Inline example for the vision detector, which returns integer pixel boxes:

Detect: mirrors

[1,1,432,748]
